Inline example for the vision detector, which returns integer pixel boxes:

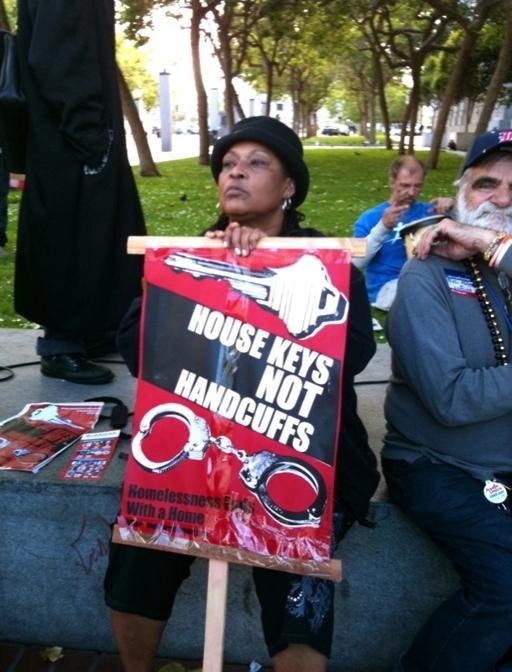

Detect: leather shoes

[40,352,115,386]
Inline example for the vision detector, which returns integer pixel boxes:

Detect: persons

[15,0,148,385]
[350,154,457,313]
[381,129,512,672]
[104,116,381,672]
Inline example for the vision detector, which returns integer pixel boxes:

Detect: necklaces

[466,253,509,367]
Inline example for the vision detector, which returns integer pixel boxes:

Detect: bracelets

[483,232,511,267]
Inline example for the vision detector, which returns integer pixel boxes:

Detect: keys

[163,252,348,340]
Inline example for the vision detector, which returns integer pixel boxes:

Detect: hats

[210,116,310,210]
[461,129,512,176]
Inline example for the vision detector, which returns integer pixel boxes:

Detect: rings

[431,225,442,236]
